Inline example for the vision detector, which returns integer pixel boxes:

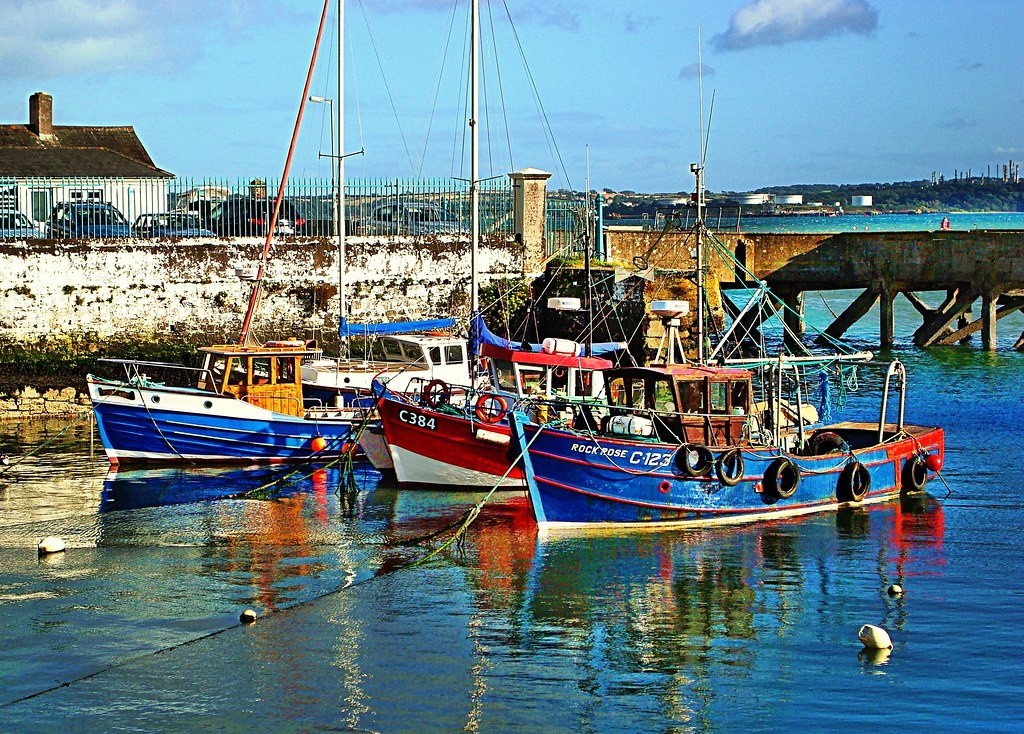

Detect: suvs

[211,195,308,237]
[44,200,135,242]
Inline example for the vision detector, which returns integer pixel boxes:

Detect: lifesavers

[770,459,800,499]
[425,379,449,408]
[475,395,507,422]
[555,369,565,378]
[905,457,928,491]
[681,443,714,477]
[425,332,451,337]
[842,462,871,502]
[717,451,745,486]
[811,432,850,454]
[267,341,305,347]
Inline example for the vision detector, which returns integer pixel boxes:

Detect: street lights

[311,95,348,357]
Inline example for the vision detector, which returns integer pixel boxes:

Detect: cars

[352,201,472,238]
[131,211,217,240]
[0,209,45,243]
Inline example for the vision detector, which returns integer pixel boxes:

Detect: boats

[257,0,509,407]
[82,0,507,471]
[373,3,820,497]
[505,85,944,538]
